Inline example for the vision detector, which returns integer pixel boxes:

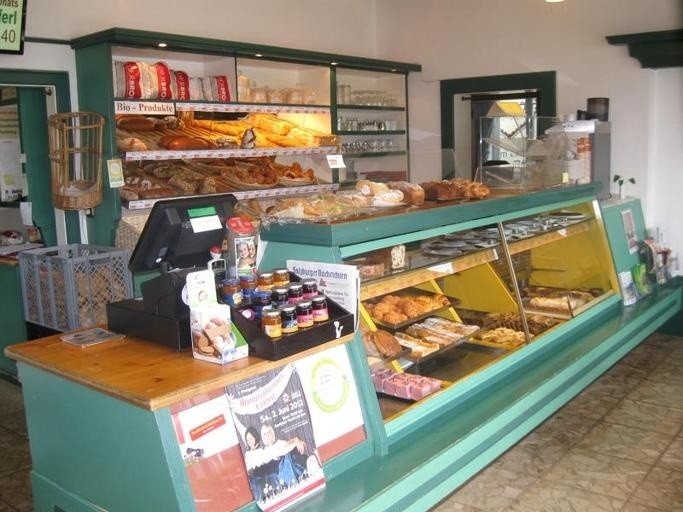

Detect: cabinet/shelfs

[259,183,625,455]
[70,27,422,245]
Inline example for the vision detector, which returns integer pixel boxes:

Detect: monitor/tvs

[128,194,238,276]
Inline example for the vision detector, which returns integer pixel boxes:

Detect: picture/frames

[0,1,28,55]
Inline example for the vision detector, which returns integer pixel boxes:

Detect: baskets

[219,170,279,190]
[18,244,133,336]
[278,176,317,187]
[184,159,229,175]
[116,214,147,252]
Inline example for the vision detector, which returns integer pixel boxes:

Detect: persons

[237,240,256,268]
[245,424,309,506]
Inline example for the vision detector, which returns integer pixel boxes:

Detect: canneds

[221,268,331,340]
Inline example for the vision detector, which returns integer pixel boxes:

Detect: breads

[365,286,597,400]
[113,114,489,221]
[237,75,316,106]
